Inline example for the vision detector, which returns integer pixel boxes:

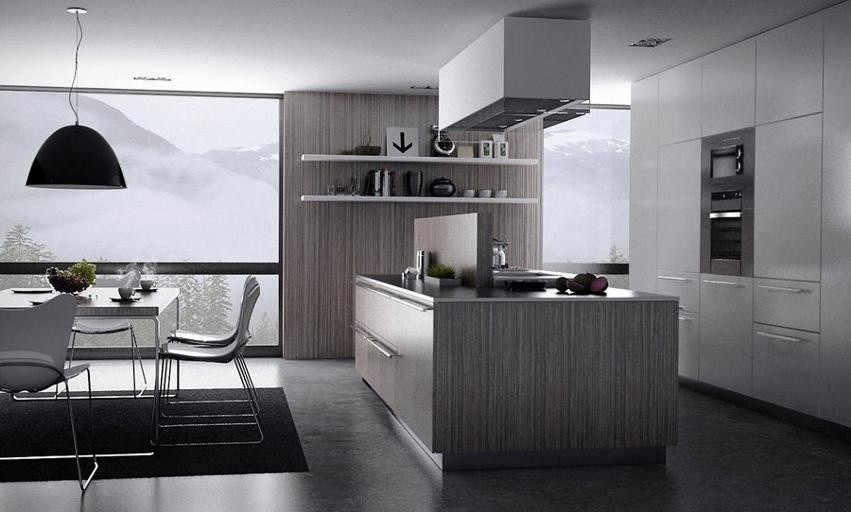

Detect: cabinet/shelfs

[629,2,851,428]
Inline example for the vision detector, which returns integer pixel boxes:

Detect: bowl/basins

[462,189,476,198]
[479,189,492,197]
[496,190,508,197]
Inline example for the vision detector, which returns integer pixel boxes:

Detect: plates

[137,289,157,292]
[11,288,54,293]
[109,297,140,302]
[30,299,81,305]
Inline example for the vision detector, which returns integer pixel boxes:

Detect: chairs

[1,294,99,492]
[70,321,146,399]
[148,286,263,447]
[161,275,261,419]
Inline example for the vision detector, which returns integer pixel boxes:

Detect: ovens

[699,128,755,276]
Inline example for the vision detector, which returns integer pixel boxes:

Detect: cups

[408,171,423,196]
[336,177,345,195]
[119,288,134,297]
[352,175,360,196]
[141,280,158,289]
[327,184,335,194]
[346,183,352,193]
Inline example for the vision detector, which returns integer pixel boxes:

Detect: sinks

[492,271,562,280]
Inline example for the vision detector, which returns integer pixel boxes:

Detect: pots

[364,169,396,197]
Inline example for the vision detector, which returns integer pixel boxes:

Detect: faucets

[493,235,510,267]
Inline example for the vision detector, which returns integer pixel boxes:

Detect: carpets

[1,386,310,480]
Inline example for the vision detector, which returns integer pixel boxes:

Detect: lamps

[25,6,129,191]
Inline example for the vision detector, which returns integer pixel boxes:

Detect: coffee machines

[491,239,509,272]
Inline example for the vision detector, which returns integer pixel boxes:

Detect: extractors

[437,16,591,143]
